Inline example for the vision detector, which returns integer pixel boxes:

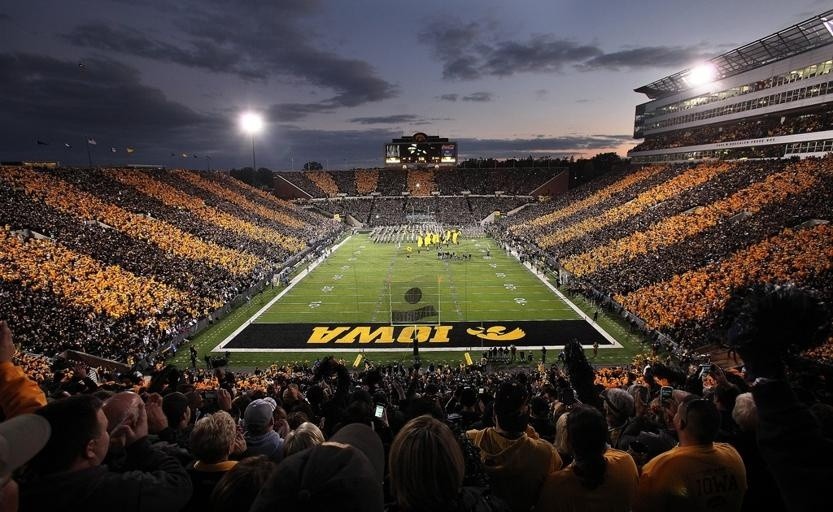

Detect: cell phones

[660,386,672,406]
[373,402,386,419]
[478,388,484,394]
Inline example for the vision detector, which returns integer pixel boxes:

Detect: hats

[251,423,385,512]
[244,396,277,427]
[0,413,50,483]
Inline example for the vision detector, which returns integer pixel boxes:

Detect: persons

[0,158,296,359]
[285,155,490,266]
[0,359,832,511]
[489,61,829,360]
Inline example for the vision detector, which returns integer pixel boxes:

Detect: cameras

[204,390,217,400]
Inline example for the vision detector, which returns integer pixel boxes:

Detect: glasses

[600,390,619,413]
[680,398,712,430]
